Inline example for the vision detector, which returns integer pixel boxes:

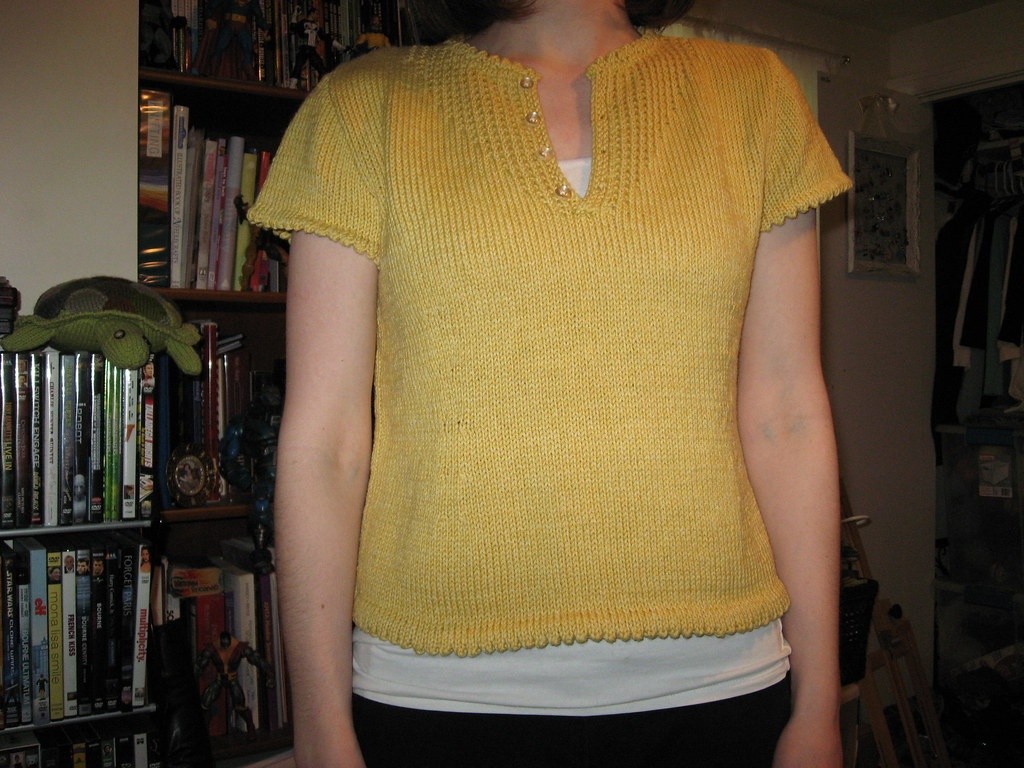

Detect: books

[137,0,403,293]
[0,316,292,768]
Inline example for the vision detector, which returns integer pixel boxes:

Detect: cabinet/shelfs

[0,274,171,768]
[138,0,404,768]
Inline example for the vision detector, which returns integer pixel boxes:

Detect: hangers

[961,140,1022,225]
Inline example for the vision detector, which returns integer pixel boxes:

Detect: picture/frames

[846,129,923,279]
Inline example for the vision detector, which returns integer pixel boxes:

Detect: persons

[48,567,61,584]
[14,755,23,768]
[193,631,276,742]
[76,558,91,577]
[244,0,854,768]
[289,5,346,90]
[140,546,151,573]
[64,555,74,574]
[234,194,289,292]
[205,0,272,81]
[354,15,391,53]
[139,0,187,68]
[92,558,104,576]
[143,361,155,388]
[36,674,47,700]
[73,474,86,524]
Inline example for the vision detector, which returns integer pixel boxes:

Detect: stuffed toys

[1,275,203,376]
